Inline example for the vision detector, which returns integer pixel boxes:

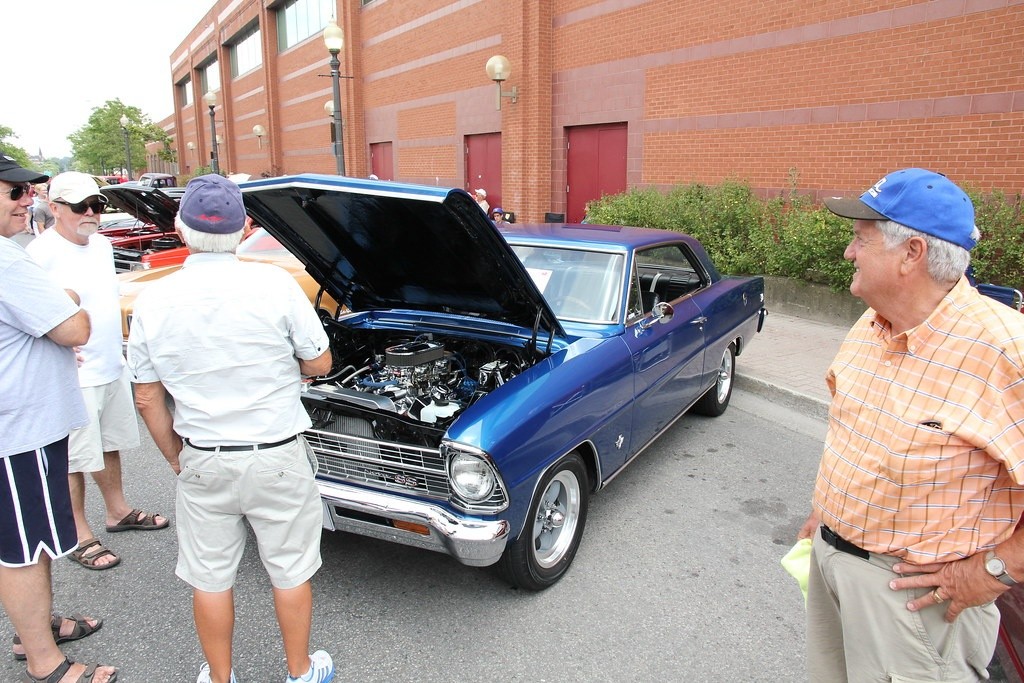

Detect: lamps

[323,100,336,121]
[252,124,266,150]
[216,135,223,154]
[187,141,196,156]
[486,55,517,111]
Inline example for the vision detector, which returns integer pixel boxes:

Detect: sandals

[11,611,104,660]
[24,654,117,683]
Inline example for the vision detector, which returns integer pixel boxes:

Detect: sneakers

[285,649,335,683]
[196,661,237,683]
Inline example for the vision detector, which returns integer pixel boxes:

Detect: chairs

[545,211,569,224]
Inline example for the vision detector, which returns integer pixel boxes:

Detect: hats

[0,153,49,184]
[49,171,110,205]
[474,188,486,196]
[822,167,977,253]
[492,208,504,215]
[178,173,246,235]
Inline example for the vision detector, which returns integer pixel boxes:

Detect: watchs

[984,550,1020,586]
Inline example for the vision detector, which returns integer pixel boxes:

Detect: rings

[932,588,944,604]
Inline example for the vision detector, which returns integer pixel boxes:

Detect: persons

[25,177,55,238]
[127,173,332,683]
[0,153,118,683]
[26,171,169,571]
[472,188,510,225]
[796,165,1024,683]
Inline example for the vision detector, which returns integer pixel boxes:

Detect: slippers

[105,507,169,533]
[67,537,122,571]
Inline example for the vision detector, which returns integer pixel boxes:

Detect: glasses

[53,200,106,214]
[0,184,31,200]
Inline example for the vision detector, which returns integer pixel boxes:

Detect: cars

[116,226,352,359]
[90,169,261,273]
[232,171,770,593]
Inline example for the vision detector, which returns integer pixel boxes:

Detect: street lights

[320,17,354,176]
[119,115,133,180]
[203,90,221,173]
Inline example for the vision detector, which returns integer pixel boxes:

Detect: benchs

[546,264,697,320]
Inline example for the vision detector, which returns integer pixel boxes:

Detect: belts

[819,523,869,560]
[184,432,300,451]
